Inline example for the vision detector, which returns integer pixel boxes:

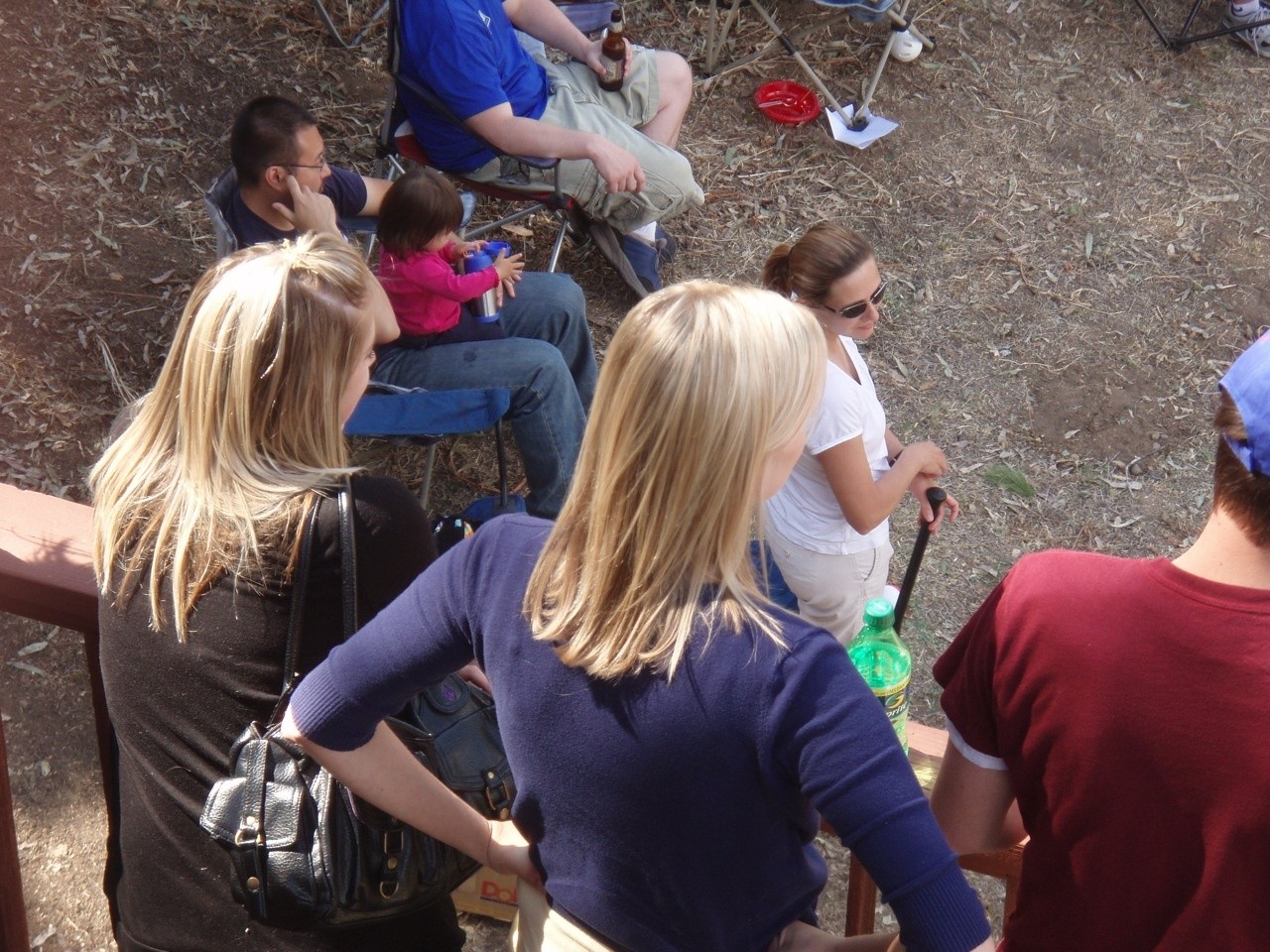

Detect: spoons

[758,98,798,107]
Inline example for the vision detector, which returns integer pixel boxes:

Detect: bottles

[846,598,913,755]
[464,239,510,324]
[598,9,626,92]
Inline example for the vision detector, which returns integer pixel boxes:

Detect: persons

[398,0,705,299]
[375,166,525,350]
[281,280,997,952]
[759,220,959,650]
[226,97,596,521]
[928,326,1270,952]
[89,228,492,952]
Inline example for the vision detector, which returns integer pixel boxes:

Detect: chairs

[205,0,937,522]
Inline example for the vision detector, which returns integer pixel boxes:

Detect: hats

[1214,326,1270,476]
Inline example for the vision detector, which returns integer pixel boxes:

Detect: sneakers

[655,229,674,262]
[589,219,665,297]
[1222,1,1270,57]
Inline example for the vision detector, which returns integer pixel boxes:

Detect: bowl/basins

[756,81,819,126]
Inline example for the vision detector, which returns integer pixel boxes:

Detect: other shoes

[881,584,899,610]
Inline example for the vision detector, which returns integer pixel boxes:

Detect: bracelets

[889,449,903,467]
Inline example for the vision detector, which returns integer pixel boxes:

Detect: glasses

[264,150,330,174]
[812,279,888,319]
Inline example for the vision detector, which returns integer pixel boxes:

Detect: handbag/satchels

[199,472,517,930]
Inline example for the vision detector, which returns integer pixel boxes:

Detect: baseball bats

[893,483,949,635]
